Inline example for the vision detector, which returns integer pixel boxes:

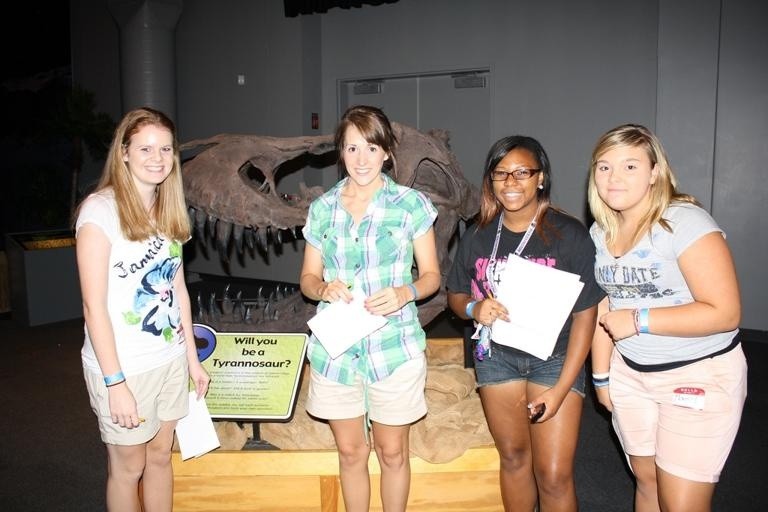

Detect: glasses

[489,167,542,182]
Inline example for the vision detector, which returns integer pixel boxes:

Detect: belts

[621,332,743,372]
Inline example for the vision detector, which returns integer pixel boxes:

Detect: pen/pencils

[487,293,505,316]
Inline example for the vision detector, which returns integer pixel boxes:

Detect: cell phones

[530,402,545,424]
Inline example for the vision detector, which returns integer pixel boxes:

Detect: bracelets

[465,300,478,319]
[632,308,648,338]
[592,372,610,387]
[407,283,417,302]
[104,371,127,387]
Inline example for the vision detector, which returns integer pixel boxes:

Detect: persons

[74,105,211,512]
[445,133,595,512]
[299,104,439,512]
[581,123,748,512]
